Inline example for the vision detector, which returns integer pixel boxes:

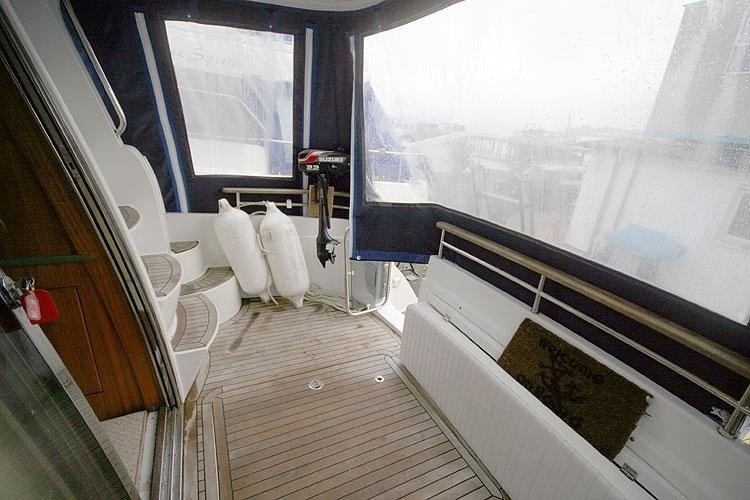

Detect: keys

[14,274,35,290]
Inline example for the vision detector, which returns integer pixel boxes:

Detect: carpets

[99,411,147,490]
